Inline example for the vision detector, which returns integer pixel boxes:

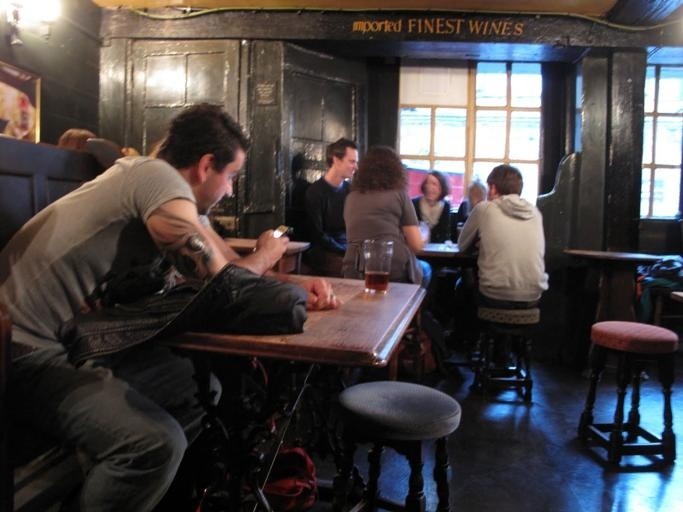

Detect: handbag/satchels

[226,446,317,510]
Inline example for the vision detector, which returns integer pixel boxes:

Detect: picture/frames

[0,60,42,144]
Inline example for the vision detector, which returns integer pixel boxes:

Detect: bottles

[420,221,429,248]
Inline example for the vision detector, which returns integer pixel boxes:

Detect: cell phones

[252,224,294,252]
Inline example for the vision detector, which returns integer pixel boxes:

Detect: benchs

[0,134,231,511]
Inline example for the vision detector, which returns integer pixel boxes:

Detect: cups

[444,240,452,249]
[361,238,392,294]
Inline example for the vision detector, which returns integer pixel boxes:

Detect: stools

[575,318,680,474]
[389,305,438,388]
[338,380,462,512]
[470,306,540,405]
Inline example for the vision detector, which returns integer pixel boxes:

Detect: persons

[429,166,547,378]
[411,171,451,243]
[339,145,434,288]
[0,102,342,512]
[304,138,359,277]
[452,181,488,228]
